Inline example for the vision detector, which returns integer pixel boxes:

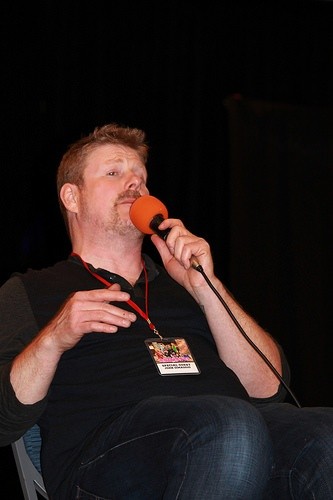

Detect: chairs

[11,424,48,500]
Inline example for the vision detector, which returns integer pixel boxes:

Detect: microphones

[128,195,203,273]
